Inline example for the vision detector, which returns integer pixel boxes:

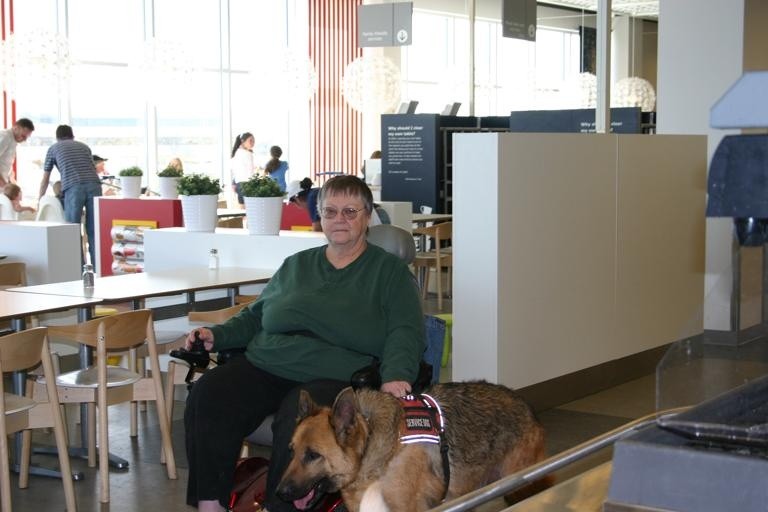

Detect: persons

[0,118,103,274]
[185,175,430,512]
[166,157,183,172]
[230,132,289,205]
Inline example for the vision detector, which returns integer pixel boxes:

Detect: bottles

[209,249,221,271]
[82,264,95,290]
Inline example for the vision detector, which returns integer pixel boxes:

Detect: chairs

[0,193,36,221]
[216,201,246,228]
[35,195,65,223]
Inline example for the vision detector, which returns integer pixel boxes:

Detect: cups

[420,205,434,215]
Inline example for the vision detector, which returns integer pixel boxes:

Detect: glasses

[319,207,366,220]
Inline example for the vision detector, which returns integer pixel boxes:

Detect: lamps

[341,1,403,118]
[609,16,656,113]
[561,8,597,110]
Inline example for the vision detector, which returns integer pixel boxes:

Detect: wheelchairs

[170,225,435,512]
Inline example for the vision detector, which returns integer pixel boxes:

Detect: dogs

[274,379,560,512]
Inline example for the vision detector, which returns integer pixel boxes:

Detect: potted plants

[156,164,184,200]
[239,171,288,235]
[118,166,144,199]
[178,172,222,233]
